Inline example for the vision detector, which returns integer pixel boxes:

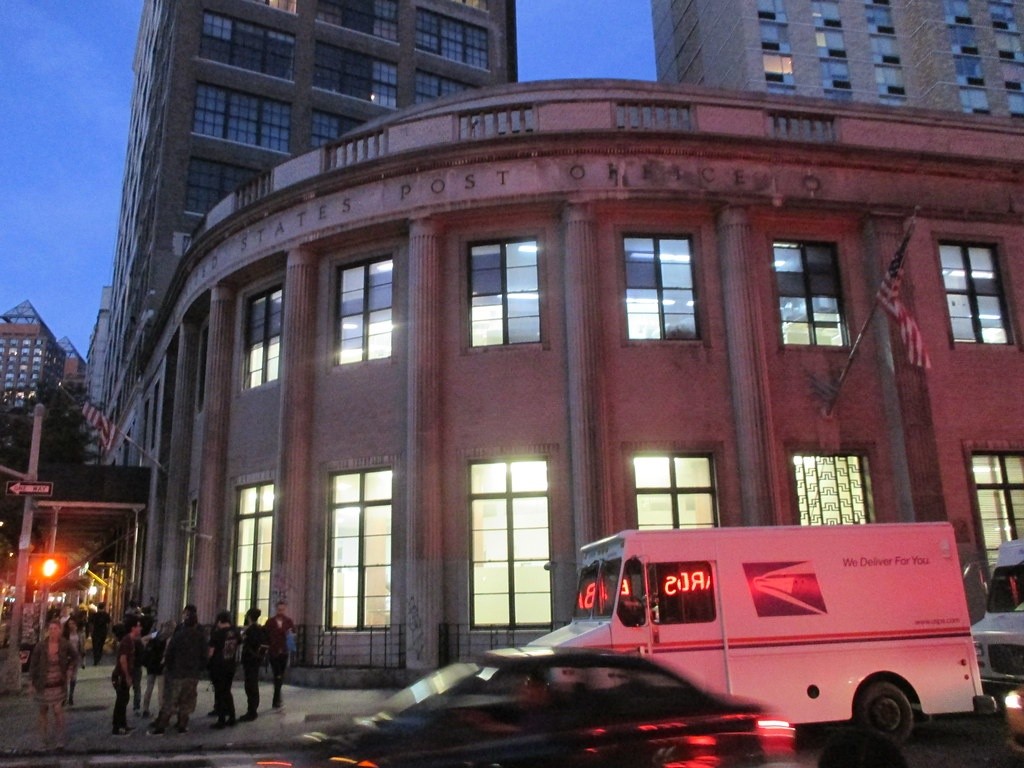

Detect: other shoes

[112,730,130,738]
[147,730,164,737]
[81,664,85,669]
[134,709,141,716]
[178,728,189,736]
[238,712,258,721]
[143,711,155,719]
[210,723,225,730]
[57,742,64,749]
[209,711,219,715]
[38,743,46,751]
[226,719,236,727]
[126,727,137,732]
[274,705,286,713]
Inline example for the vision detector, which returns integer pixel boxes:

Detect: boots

[69,680,75,704]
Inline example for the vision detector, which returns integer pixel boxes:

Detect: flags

[75,391,117,452]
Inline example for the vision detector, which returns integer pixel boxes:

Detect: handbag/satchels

[140,647,155,670]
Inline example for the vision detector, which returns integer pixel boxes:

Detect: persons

[110,599,158,735]
[207,611,239,730]
[140,619,177,718]
[47,597,97,653]
[240,608,264,722]
[465,679,545,732]
[29,620,82,751]
[876,217,931,370]
[62,617,87,706]
[89,601,112,666]
[146,605,204,735]
[263,600,299,714]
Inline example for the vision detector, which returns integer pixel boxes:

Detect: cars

[296,647,799,767]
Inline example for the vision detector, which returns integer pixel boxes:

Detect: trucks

[965,537,1024,707]
[527,520,985,747]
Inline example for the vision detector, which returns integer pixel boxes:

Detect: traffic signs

[5,480,55,497]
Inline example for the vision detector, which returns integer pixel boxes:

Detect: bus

[0,579,15,647]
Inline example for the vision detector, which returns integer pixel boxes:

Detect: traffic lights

[38,556,65,584]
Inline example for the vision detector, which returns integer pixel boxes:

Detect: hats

[185,604,196,612]
[129,600,138,610]
